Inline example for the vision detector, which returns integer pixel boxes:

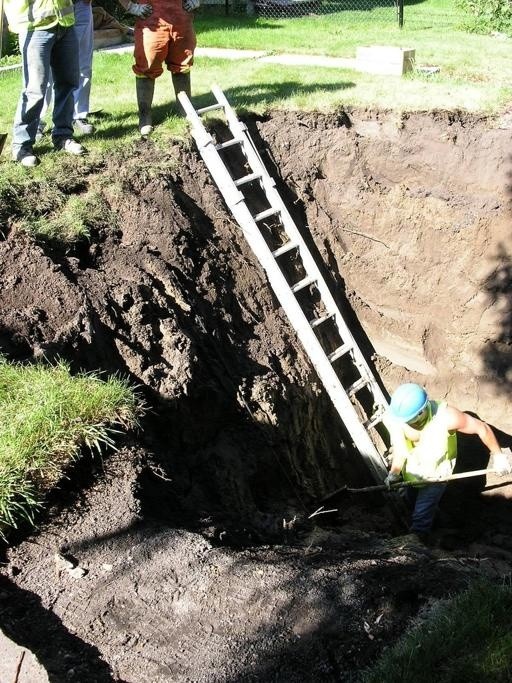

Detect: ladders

[178,80,418,529]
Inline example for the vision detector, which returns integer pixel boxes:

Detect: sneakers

[75,120,94,134]
[19,153,38,167]
[139,123,153,135]
[59,139,85,155]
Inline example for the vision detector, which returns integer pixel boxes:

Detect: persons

[3,0,84,167]
[118,0,203,136]
[37,0,94,135]
[384,383,512,546]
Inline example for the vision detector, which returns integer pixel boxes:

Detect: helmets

[390,381,428,422]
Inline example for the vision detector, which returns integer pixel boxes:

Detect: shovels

[319,469,497,502]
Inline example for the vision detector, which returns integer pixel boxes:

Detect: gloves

[182,0,200,11]
[383,473,404,496]
[125,1,152,20]
[493,453,512,477]
